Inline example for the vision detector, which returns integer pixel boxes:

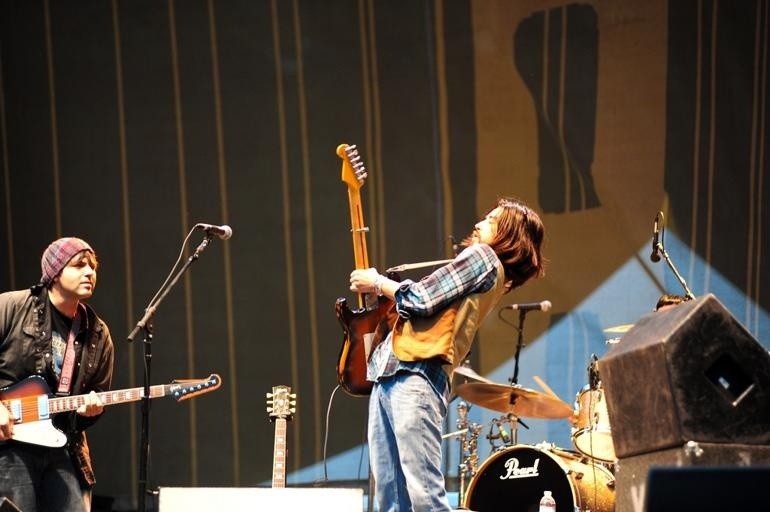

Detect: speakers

[614,441,769,512]
[596,290,769,459]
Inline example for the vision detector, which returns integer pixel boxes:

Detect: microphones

[496,419,511,443]
[198,223,234,240]
[650,216,661,263]
[508,299,552,312]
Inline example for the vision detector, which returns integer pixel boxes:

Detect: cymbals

[451,366,490,383]
[603,322,634,335]
[450,380,574,421]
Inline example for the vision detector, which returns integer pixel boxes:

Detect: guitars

[265,385,297,488]
[333,143,399,399]
[0,374,222,448]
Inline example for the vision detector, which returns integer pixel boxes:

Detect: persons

[1,237,115,511]
[350,196,550,512]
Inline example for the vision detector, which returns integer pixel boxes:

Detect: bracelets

[374,274,388,297]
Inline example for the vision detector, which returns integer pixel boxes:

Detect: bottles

[539,490,556,512]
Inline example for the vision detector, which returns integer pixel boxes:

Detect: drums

[459,444,618,512]
[572,383,620,464]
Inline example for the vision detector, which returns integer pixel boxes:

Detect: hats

[41,237,94,284]
